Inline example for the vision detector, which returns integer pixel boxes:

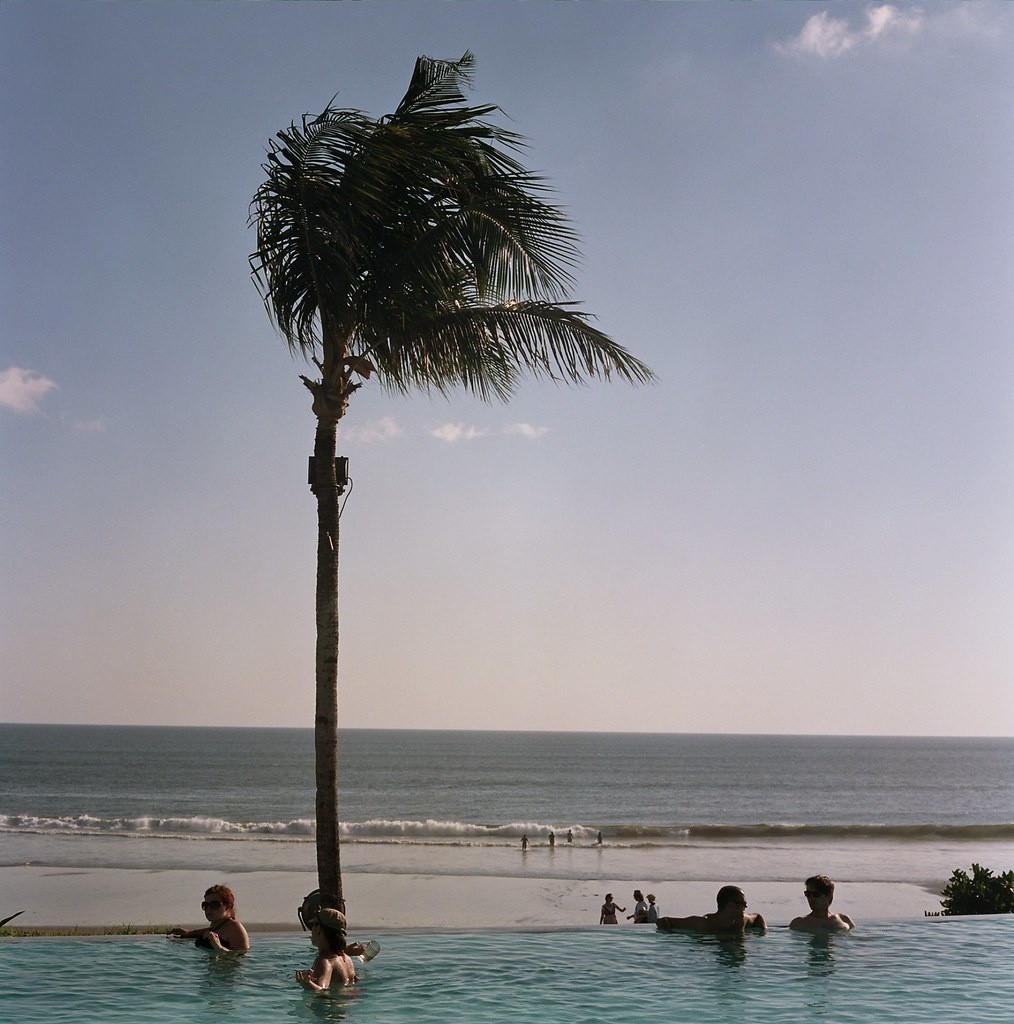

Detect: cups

[358,940,380,965]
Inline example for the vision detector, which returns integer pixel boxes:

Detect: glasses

[739,901,746,907]
[202,900,225,910]
[804,890,824,897]
[609,897,613,899]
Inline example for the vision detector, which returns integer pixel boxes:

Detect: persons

[548,831,555,845]
[655,885,768,934]
[520,834,529,850]
[567,830,574,843]
[598,831,602,844]
[626,890,660,924]
[789,876,856,931]
[296,894,366,989]
[600,893,626,924]
[167,884,250,951]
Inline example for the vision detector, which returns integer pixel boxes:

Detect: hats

[647,894,655,903]
[308,907,347,936]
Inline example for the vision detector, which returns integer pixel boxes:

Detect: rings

[215,934,218,937]
